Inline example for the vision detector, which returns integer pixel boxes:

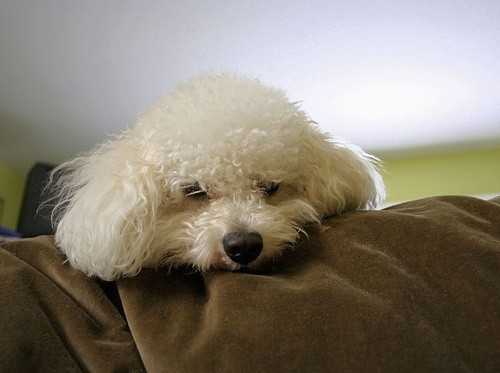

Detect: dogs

[35,71,388,281]
